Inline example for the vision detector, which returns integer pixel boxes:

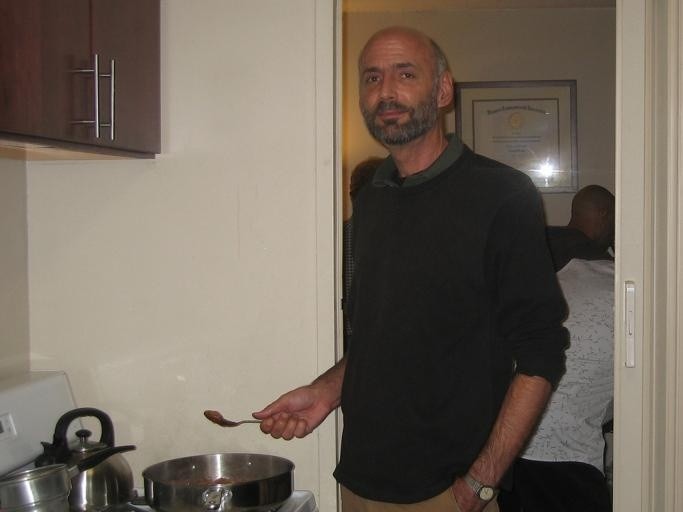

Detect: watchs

[463,473,501,504]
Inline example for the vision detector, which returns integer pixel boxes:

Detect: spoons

[202,409,262,427]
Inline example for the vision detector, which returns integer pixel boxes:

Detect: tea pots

[35,407,137,511]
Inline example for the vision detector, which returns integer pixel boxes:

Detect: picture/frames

[454,80,578,193]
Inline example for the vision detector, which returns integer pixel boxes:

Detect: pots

[0,445,136,512]
[142,452,296,512]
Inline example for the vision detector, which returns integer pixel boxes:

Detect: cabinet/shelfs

[0,0,161,158]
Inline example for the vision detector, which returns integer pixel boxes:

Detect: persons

[494,256,616,512]
[545,185,615,274]
[251,26,571,512]
